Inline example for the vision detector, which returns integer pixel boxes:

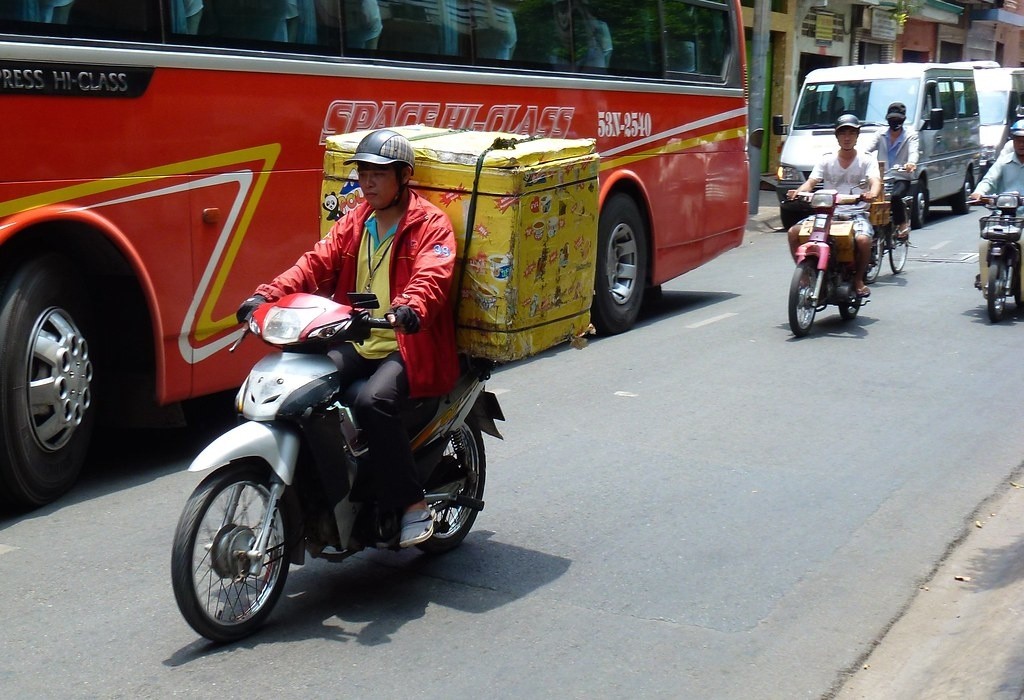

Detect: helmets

[343,129,415,176]
[834,114,862,133]
[1010,120,1024,136]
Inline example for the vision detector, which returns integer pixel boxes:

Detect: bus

[0,0,750,510]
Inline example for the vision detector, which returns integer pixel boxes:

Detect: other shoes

[974,273,982,288]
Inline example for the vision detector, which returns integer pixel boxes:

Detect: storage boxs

[798,218,857,263]
[320,124,600,362]
[869,160,891,225]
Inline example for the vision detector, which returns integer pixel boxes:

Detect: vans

[772,61,1024,233]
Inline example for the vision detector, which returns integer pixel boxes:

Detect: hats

[887,102,906,119]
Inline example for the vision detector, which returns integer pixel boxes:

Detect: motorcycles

[780,179,871,337]
[965,191,1024,322]
[169,292,505,644]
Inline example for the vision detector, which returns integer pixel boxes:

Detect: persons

[237,131,457,546]
[970,118,1024,289]
[787,114,883,297]
[864,102,920,238]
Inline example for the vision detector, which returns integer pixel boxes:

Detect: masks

[888,119,904,131]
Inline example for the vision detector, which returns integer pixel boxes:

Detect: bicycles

[862,165,914,284]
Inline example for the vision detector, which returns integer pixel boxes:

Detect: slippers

[856,286,871,297]
[895,227,911,239]
[399,508,437,548]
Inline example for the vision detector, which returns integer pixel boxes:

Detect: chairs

[823,97,846,120]
[0,0,729,77]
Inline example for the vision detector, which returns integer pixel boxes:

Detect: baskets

[979,216,1024,241]
